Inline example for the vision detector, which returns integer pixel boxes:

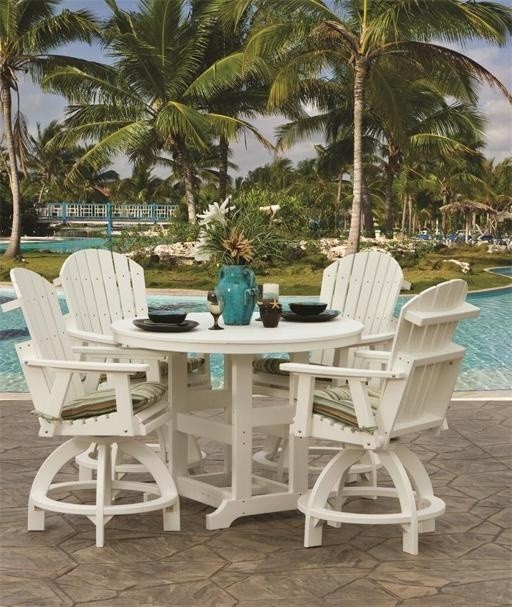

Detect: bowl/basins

[148,310,188,324]
[289,302,327,316]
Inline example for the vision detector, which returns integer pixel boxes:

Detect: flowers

[193,194,286,267]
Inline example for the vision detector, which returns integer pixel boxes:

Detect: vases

[215,266,259,325]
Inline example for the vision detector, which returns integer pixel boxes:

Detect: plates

[280,310,340,322]
[132,318,200,333]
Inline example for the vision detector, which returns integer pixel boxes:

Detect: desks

[111,312,365,531]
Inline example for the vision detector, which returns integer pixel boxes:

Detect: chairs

[252,250,411,500]
[53,247,207,501]
[279,279,464,553]
[10,266,179,548]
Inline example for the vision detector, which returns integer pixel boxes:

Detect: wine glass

[207,290,225,330]
[255,285,263,321]
